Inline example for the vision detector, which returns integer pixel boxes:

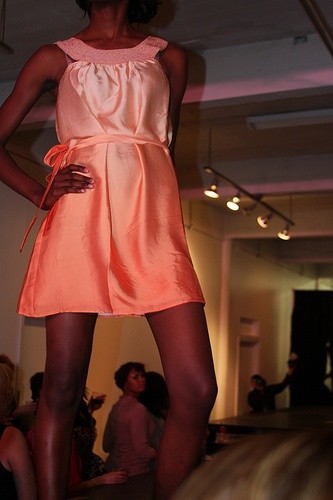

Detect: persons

[248,356,298,413]
[0,353,41,500]
[0,0,219,500]
[3,355,230,500]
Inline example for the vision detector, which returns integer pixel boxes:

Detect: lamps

[256,214,274,228]
[203,176,219,199]
[242,205,259,217]
[226,190,241,211]
[278,223,291,240]
